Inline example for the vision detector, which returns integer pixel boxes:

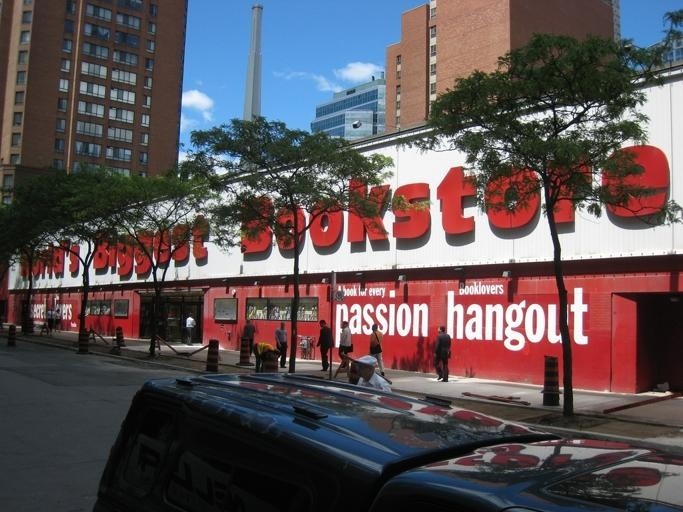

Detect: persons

[47,307,55,336]
[317,320,332,371]
[185,312,196,345]
[369,324,385,377]
[339,321,352,368]
[243,318,254,356]
[55,309,62,330]
[275,321,287,362]
[432,326,451,382]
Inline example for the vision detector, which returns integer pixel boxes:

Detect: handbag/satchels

[448,352,451,359]
[344,343,353,354]
[370,344,383,355]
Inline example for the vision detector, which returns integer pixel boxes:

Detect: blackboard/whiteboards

[114,299,128,316]
[214,298,238,321]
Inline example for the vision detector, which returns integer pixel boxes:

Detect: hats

[353,354,379,368]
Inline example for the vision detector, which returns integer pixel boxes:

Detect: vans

[91,371,683,512]
